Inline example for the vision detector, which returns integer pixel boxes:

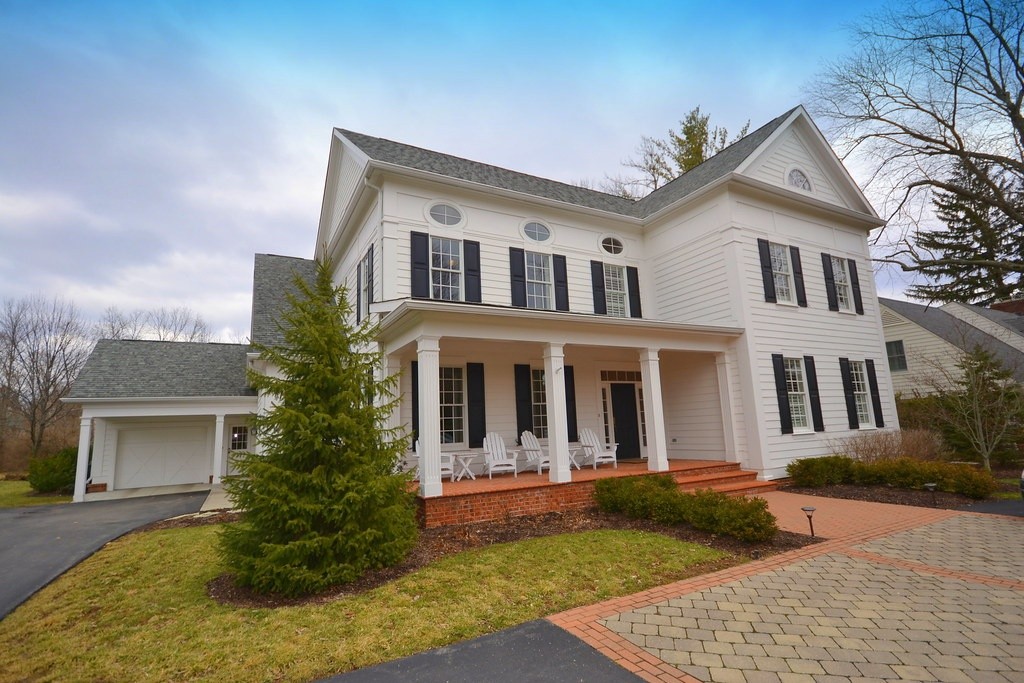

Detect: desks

[568,447,583,470]
[452,452,478,481]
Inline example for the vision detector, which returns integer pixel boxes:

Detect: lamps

[925,482,938,492]
[801,506,816,517]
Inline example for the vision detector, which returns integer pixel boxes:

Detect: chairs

[579,427,620,470]
[519,430,551,476]
[480,432,519,479]
[411,439,452,481]
